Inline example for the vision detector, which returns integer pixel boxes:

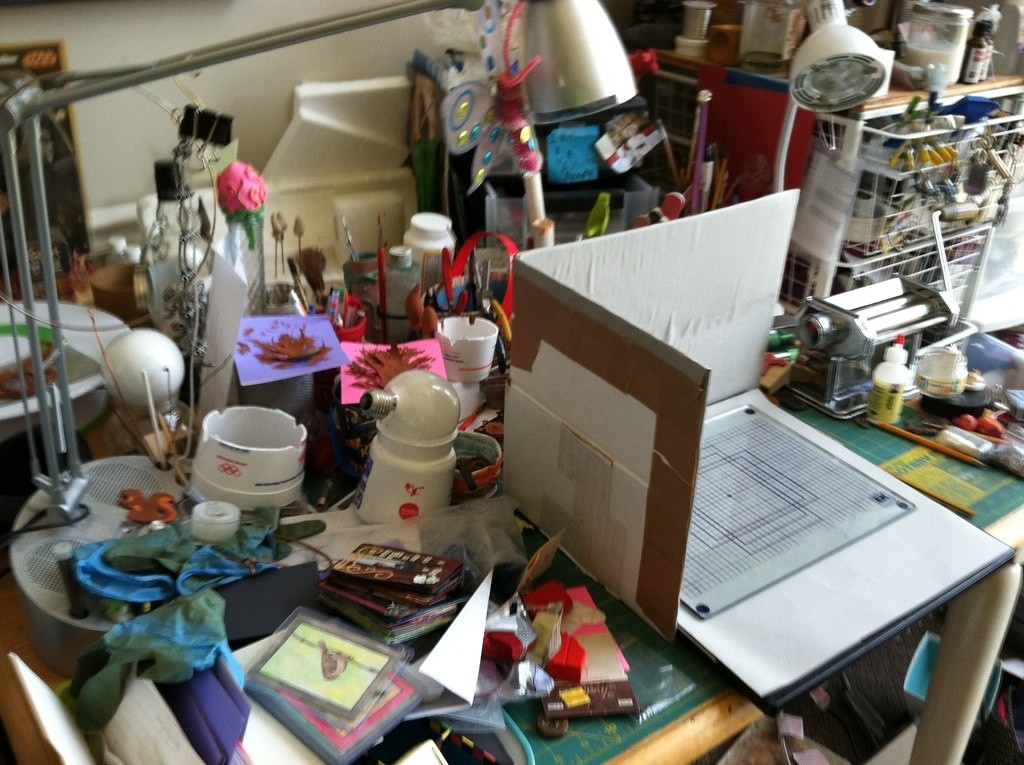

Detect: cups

[344,243,382,307]
[901,2,976,85]
[316,308,368,342]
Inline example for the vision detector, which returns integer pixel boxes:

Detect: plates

[0,299,129,419]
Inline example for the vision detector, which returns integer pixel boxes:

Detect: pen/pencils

[283,254,367,332]
[868,417,990,468]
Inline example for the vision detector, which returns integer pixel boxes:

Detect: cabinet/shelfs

[647,39,1024,318]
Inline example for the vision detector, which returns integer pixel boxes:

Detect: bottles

[382,246,419,318]
[98,236,145,270]
[736,0,803,76]
[867,334,911,426]
[148,158,209,325]
[403,212,456,285]
[958,20,994,84]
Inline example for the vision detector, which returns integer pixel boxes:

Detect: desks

[0,389,1022,765]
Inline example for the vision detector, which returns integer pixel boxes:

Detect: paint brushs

[340,215,357,260]
[375,214,388,343]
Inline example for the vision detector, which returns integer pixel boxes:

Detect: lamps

[0,1,638,677]
[773,0,890,327]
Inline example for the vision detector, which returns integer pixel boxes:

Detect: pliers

[440,246,469,314]
[473,258,511,373]
